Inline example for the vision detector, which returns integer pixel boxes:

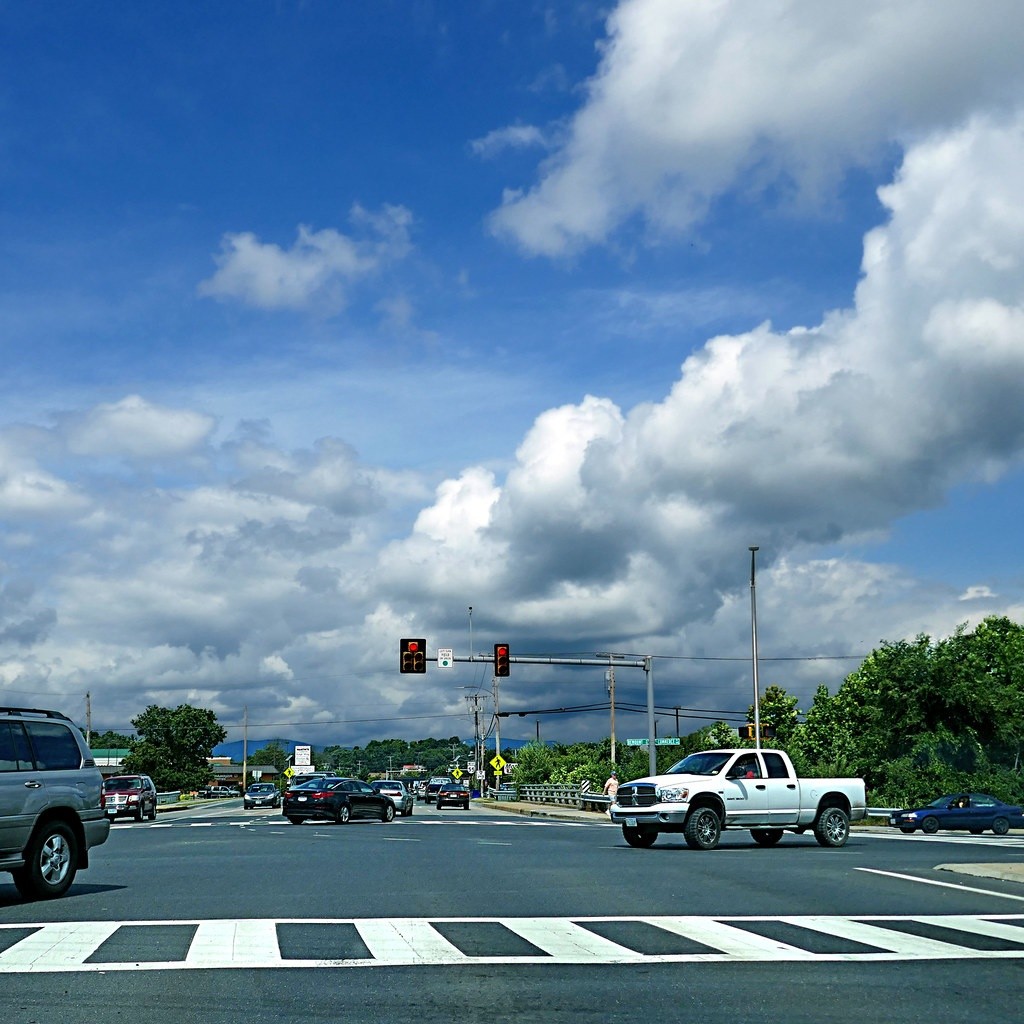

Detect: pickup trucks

[197,785,240,798]
[609,749,869,850]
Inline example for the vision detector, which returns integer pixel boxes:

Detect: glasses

[738,763,745,766]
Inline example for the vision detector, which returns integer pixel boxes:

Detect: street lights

[748,545,761,749]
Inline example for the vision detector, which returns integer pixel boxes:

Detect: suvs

[0,706,110,900]
[103,773,158,823]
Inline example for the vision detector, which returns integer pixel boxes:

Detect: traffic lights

[738,726,749,737]
[494,643,511,677]
[763,726,775,738]
[400,638,427,674]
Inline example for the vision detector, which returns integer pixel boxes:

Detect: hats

[611,771,617,774]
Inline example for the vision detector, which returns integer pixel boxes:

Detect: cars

[370,779,414,817]
[888,792,1024,837]
[244,783,282,810]
[288,772,337,791]
[416,778,470,811]
[282,777,397,825]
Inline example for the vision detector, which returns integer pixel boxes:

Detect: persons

[603,772,619,816]
[958,798,964,807]
[740,761,753,778]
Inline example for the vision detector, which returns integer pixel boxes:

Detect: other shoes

[605,811,611,816]
